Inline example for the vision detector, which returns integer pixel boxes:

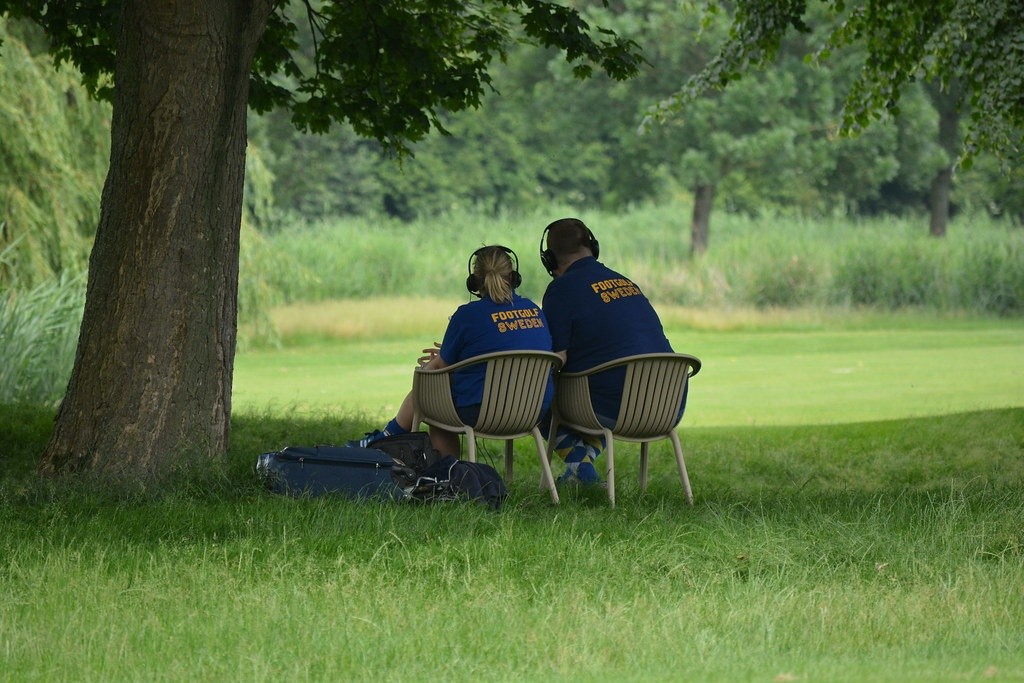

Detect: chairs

[540,352,701,509]
[412,350,564,505]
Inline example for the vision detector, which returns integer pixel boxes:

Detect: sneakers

[345,429,380,448]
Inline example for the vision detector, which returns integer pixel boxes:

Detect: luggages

[253,447,418,506]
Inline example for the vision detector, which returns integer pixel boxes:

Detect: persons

[345,245,555,461]
[537,218,688,508]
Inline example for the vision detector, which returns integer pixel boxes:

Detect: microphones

[470,290,482,298]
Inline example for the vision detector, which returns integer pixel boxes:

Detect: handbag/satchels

[433,460,507,514]
[408,481,453,504]
[365,431,442,479]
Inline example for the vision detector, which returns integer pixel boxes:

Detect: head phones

[540,218,599,271]
[467,245,521,292]
